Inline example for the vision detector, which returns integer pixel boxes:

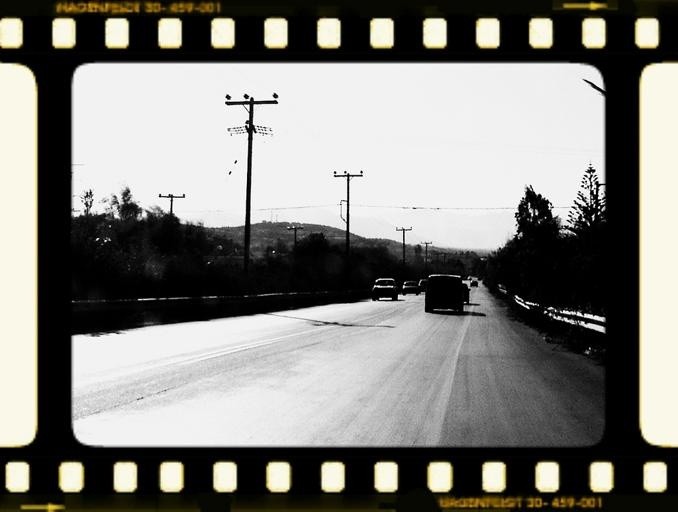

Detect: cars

[367,267,483,316]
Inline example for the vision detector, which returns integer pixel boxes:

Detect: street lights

[332,167,363,296]
[157,190,185,298]
[276,217,433,281]
[221,87,279,298]
